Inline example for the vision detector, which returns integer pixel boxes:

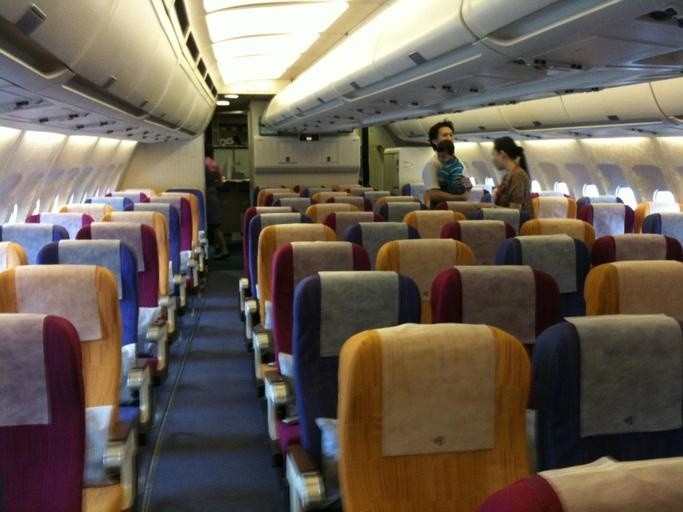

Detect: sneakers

[213,253,231,260]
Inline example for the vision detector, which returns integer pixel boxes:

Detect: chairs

[2,187,207,508]
[238,182,682,508]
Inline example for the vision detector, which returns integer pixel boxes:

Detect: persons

[422,120,473,208]
[421,139,465,208]
[491,136,534,222]
[205,143,231,259]
